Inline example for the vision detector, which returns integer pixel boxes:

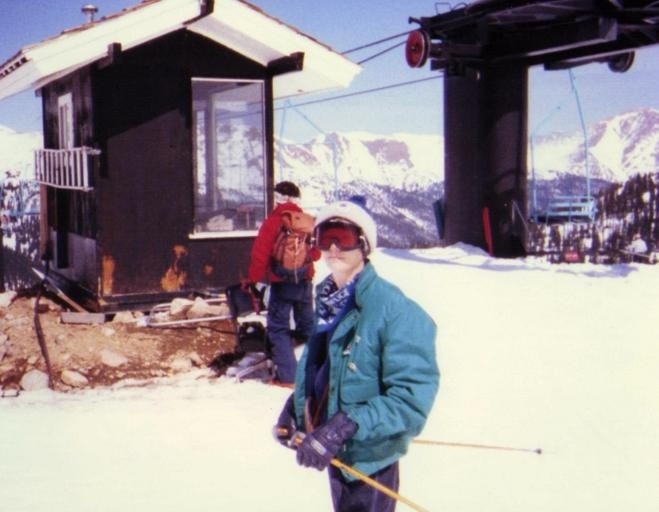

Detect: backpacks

[268,209,317,283]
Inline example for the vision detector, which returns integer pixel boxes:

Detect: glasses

[317,224,361,253]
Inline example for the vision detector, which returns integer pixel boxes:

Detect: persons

[278,200,441,512]
[241,182,321,388]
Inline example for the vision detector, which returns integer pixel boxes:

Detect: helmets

[311,201,377,256]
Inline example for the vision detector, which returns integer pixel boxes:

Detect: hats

[273,181,302,198]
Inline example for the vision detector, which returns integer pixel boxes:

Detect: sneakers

[262,377,297,390]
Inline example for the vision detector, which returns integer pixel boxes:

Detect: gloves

[275,393,303,449]
[294,411,358,472]
